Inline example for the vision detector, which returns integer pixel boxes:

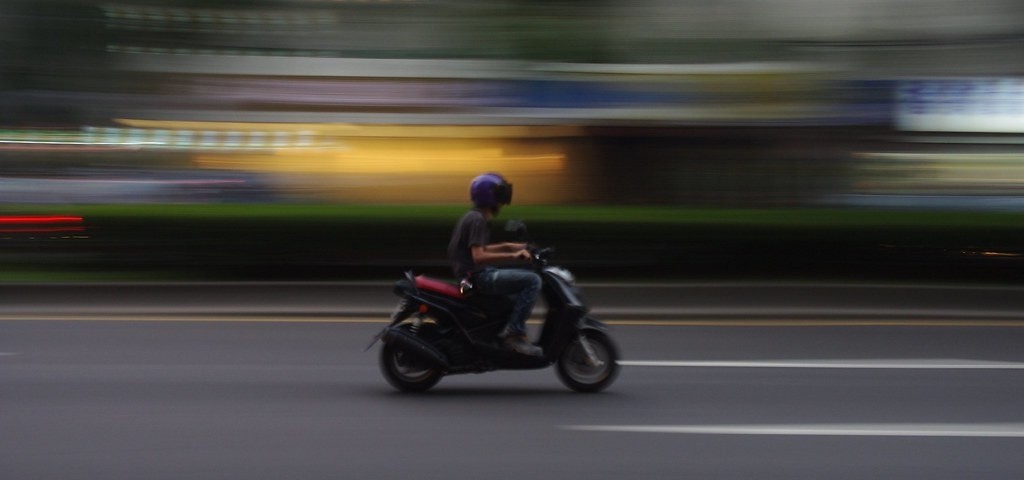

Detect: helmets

[469,174,507,203]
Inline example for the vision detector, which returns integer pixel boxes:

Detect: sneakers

[502,333,544,357]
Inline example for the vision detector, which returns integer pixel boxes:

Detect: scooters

[360,239,624,395]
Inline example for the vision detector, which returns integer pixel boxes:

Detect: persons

[449,172,542,357]
